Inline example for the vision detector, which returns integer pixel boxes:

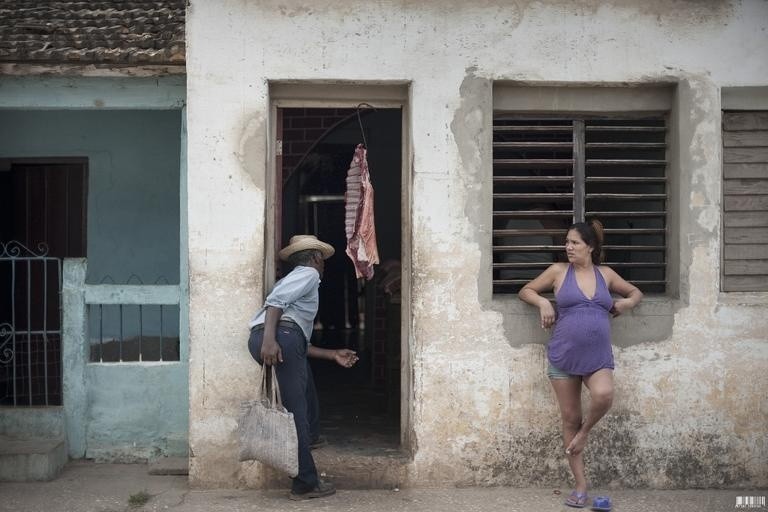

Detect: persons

[517,219,644,508]
[246,231,361,499]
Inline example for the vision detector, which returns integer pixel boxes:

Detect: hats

[278,235,335,262]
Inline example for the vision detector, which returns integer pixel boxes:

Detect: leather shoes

[288,483,336,501]
[310,435,328,448]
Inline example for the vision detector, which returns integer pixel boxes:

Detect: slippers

[566,490,588,507]
[592,496,613,511]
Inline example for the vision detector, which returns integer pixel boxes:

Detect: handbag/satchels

[237,396,300,478]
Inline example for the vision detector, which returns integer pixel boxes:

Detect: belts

[251,322,295,330]
